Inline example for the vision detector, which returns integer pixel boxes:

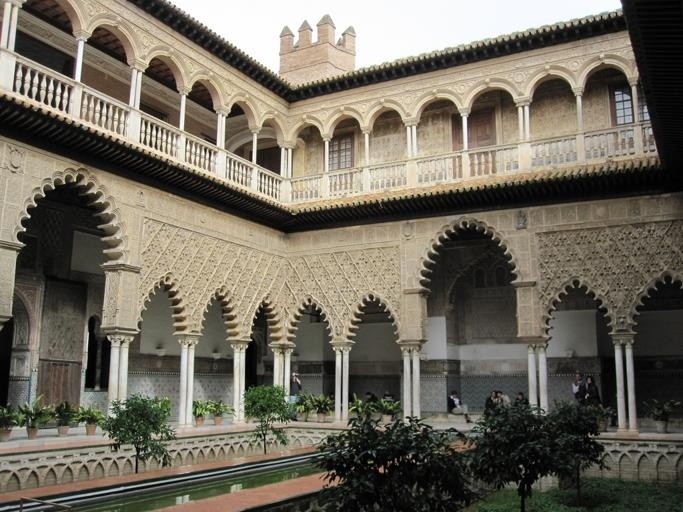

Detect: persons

[365,391,377,403]
[484,391,502,414]
[383,388,393,399]
[585,375,602,406]
[514,392,529,407]
[496,391,512,407]
[572,373,588,402]
[290,369,301,403]
[449,390,474,423]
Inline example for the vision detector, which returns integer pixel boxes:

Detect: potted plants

[642,398,681,433]
[374,397,404,423]
[593,404,611,432]
[291,391,334,423]
[192,399,237,426]
[0,394,108,443]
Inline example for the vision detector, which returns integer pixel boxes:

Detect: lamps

[212,350,221,359]
[156,347,166,357]
